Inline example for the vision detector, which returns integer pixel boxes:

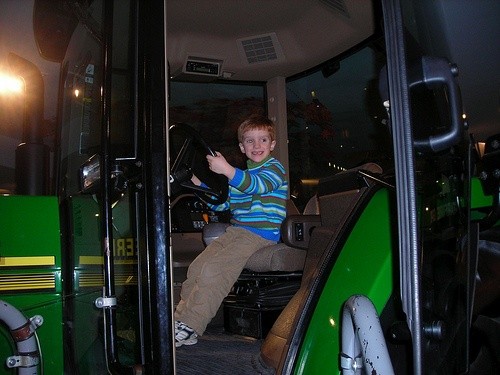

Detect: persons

[171,115,288,347]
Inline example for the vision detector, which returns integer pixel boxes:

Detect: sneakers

[174,320,197,347]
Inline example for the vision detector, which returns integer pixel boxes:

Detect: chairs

[201,162,387,371]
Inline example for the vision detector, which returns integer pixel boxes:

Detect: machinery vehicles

[1,0,499,375]
